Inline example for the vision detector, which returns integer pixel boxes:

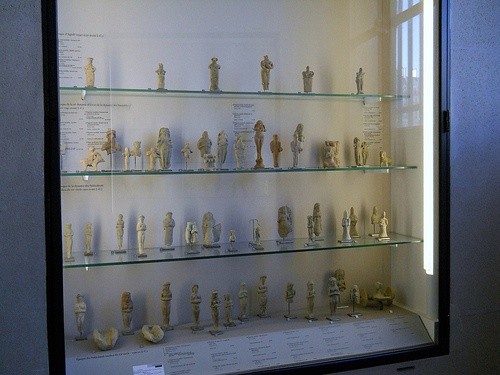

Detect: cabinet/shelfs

[40,1,452,375]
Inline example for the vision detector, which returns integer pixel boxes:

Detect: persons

[65,52,397,344]
